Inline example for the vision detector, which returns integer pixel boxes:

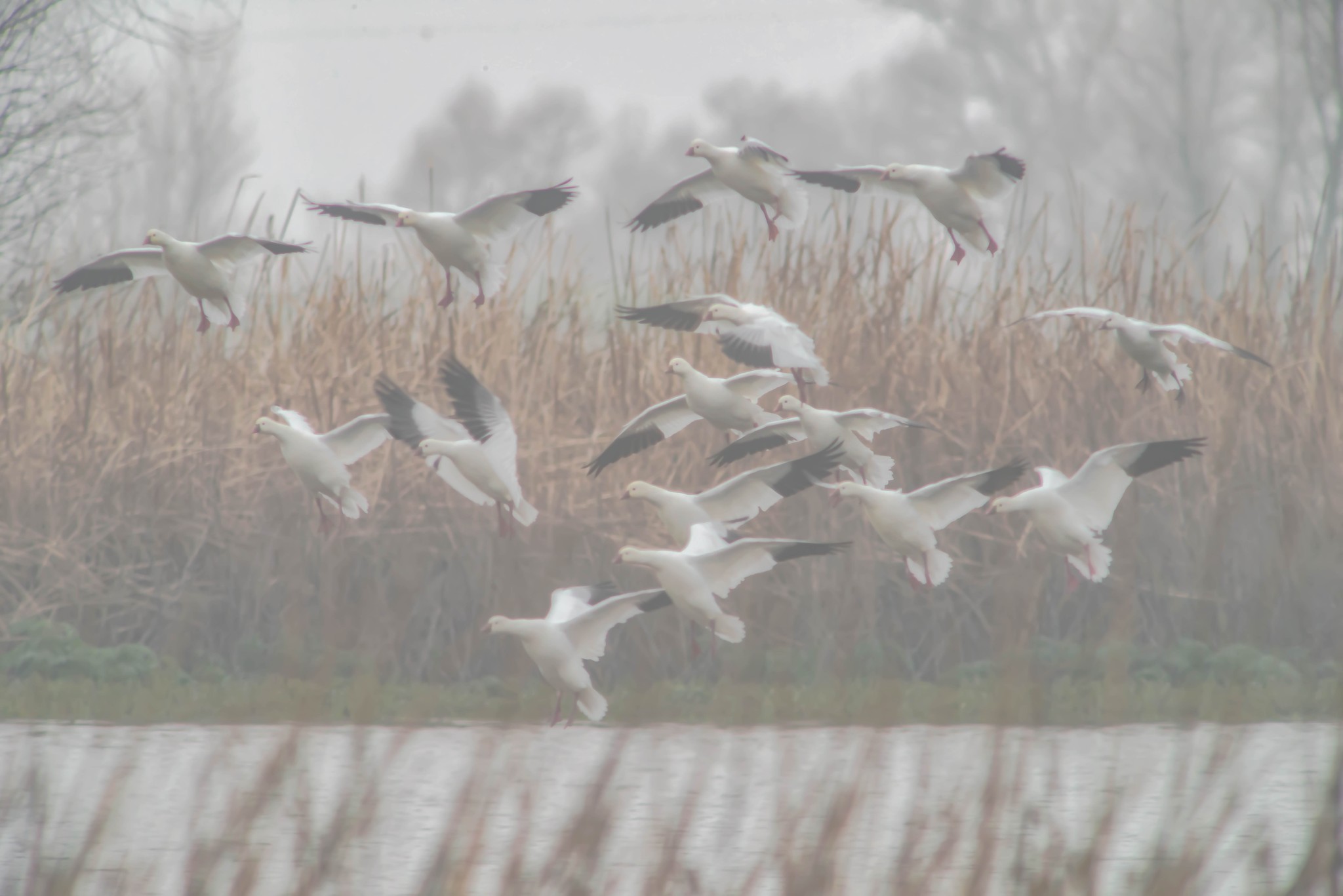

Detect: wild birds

[481,293,1206,730]
[620,136,1026,267]
[251,352,539,537]
[1004,306,1278,409]
[51,228,319,332]
[298,177,581,310]
